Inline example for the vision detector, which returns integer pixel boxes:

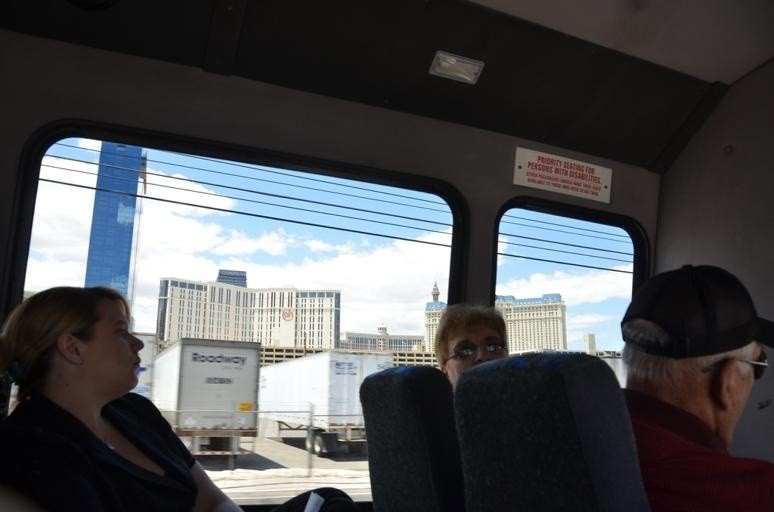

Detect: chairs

[451,348,652,511]
[357,364,464,511]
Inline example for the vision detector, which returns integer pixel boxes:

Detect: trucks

[147,336,260,464]
[127,325,165,402]
[252,349,396,460]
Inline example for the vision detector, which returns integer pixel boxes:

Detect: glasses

[704,353,768,378]
[444,336,507,362]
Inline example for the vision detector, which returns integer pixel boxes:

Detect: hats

[621,264,773,359]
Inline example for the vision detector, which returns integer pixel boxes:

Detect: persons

[0,285,357,512]
[619,264,773,511]
[433,302,509,393]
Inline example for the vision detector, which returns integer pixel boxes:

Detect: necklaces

[99,423,117,450]
